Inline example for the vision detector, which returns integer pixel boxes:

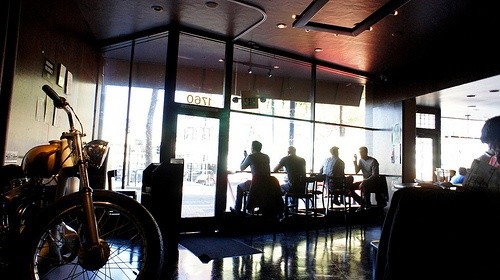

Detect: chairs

[242,175,389,219]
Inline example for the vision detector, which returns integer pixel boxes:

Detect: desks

[237,171,400,175]
[393,178,461,190]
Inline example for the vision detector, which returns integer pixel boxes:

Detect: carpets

[179,232,264,261]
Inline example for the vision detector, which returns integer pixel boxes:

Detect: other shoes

[230,206,240,216]
[288,200,296,206]
[340,200,343,203]
[355,206,371,213]
[333,199,340,205]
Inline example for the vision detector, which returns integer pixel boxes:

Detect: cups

[436,167,450,183]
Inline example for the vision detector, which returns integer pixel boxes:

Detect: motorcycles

[0,83,166,280]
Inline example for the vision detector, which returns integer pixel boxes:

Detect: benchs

[370,185,500,280]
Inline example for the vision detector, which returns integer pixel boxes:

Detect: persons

[230,140,270,213]
[274,146,306,214]
[451,167,467,185]
[348,146,379,212]
[465,115,500,189]
[322,146,354,206]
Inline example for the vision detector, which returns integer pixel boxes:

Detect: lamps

[249,64,253,74]
[233,88,266,110]
[266,68,272,77]
